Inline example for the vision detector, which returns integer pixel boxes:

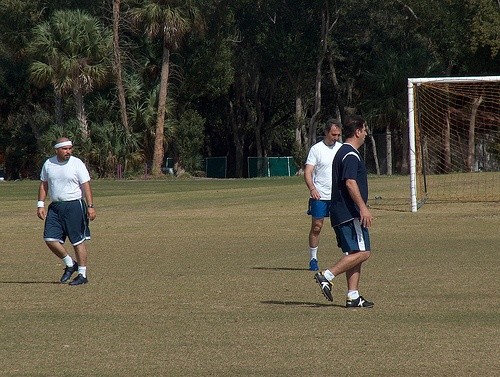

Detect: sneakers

[313,272,335,302]
[346,296,375,308]
[309,258,319,271]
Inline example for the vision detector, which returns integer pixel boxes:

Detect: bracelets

[87,204,94,208]
[37,201,44,207]
[310,188,315,192]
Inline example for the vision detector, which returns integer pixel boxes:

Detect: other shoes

[60,260,78,282]
[69,274,87,285]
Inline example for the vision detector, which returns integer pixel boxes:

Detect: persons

[303,119,343,270]
[314,117,375,308]
[172,160,180,177]
[37,137,97,285]
